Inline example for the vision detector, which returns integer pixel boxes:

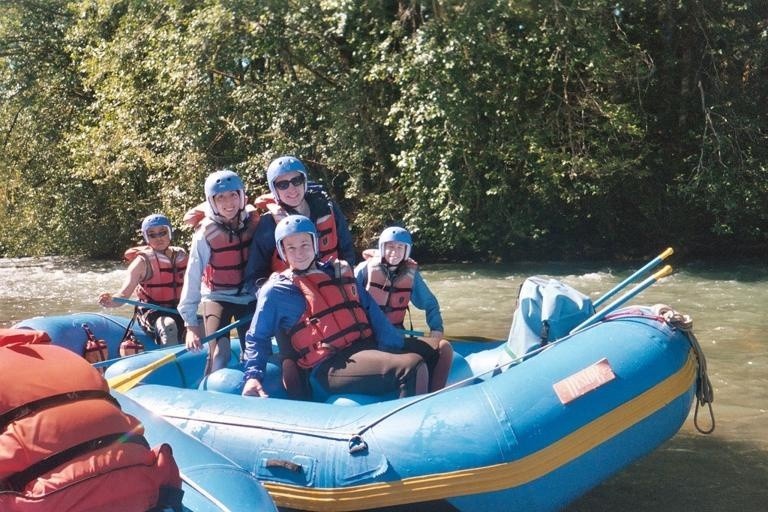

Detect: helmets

[276,215,319,260]
[268,156,308,200]
[206,170,245,213]
[378,226,412,261]
[142,215,173,243]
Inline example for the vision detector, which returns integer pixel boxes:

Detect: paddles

[106,311,255,395]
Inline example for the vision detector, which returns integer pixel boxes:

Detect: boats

[9,274,718,511]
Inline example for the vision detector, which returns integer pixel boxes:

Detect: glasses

[148,230,168,239]
[274,173,306,189]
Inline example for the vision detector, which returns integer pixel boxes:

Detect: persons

[241,216,451,400]
[176,172,260,371]
[241,156,355,293]
[356,227,443,338]
[99,213,188,346]
